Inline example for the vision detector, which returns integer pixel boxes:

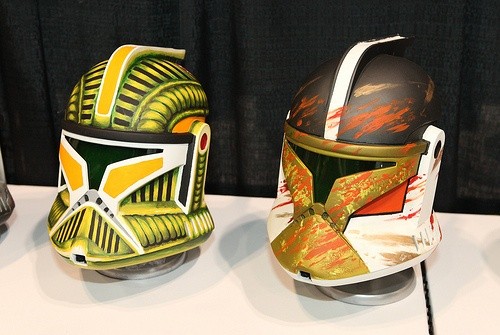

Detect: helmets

[46,45,217,270]
[266,34,445,285]
